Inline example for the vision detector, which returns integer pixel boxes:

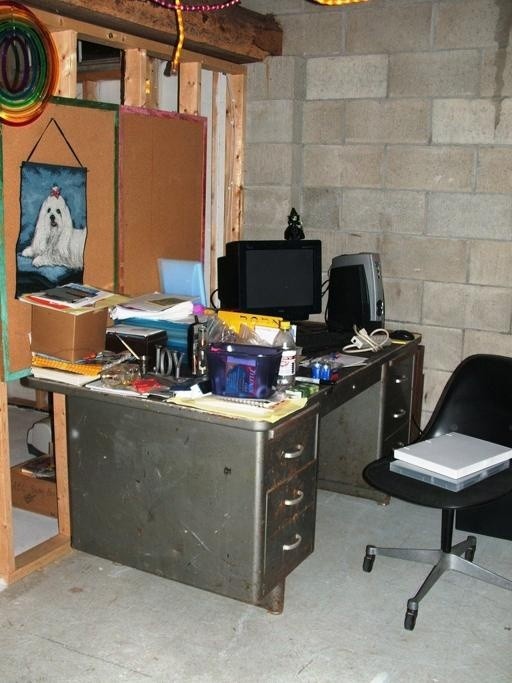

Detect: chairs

[363,354,511,629]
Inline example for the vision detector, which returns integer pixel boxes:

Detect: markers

[295,376,338,385]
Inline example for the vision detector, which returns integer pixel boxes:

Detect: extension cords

[350,332,393,349]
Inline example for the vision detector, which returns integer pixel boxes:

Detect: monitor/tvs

[217,240,322,323]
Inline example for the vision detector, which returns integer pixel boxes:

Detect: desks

[20,317,425,615]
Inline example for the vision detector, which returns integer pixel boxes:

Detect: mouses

[391,328,414,341]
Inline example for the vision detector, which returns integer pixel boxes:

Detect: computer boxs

[327,253,385,333]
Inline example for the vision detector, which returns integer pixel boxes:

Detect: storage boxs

[11,454,56,520]
[29,305,107,364]
[114,301,218,369]
[205,341,283,399]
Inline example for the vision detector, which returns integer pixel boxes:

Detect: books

[31,348,131,377]
[214,394,286,408]
[20,281,131,317]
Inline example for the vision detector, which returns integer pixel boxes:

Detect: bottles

[272,320,297,388]
[197,326,209,381]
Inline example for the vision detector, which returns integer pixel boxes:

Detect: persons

[284,205,306,241]
